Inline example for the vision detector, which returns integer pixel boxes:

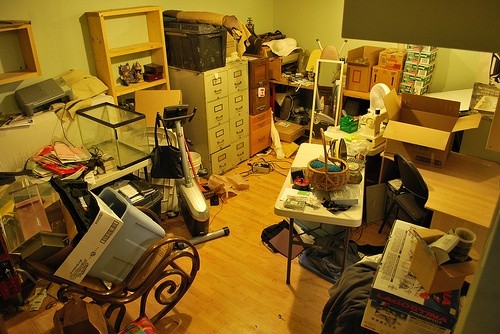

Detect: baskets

[305,127,348,193]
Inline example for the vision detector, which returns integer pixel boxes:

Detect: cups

[452,228,476,259]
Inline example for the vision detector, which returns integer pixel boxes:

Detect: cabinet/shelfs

[85,6,170,117]
[170,55,272,174]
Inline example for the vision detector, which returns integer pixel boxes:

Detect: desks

[379,141,500,258]
[84,142,152,189]
[270,78,370,116]
[274,142,364,285]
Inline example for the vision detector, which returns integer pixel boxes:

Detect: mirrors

[309,60,344,142]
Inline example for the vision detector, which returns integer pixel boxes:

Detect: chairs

[378,154,429,234]
[20,206,201,332]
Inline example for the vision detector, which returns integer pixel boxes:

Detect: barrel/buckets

[187,152,201,177]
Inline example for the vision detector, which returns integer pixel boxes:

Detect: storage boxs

[227,31,237,57]
[133,89,183,129]
[52,299,108,334]
[164,22,226,72]
[17,182,165,284]
[361,219,459,334]
[381,88,483,167]
[76,102,150,168]
[274,119,305,142]
[346,43,439,96]
[407,227,479,294]
